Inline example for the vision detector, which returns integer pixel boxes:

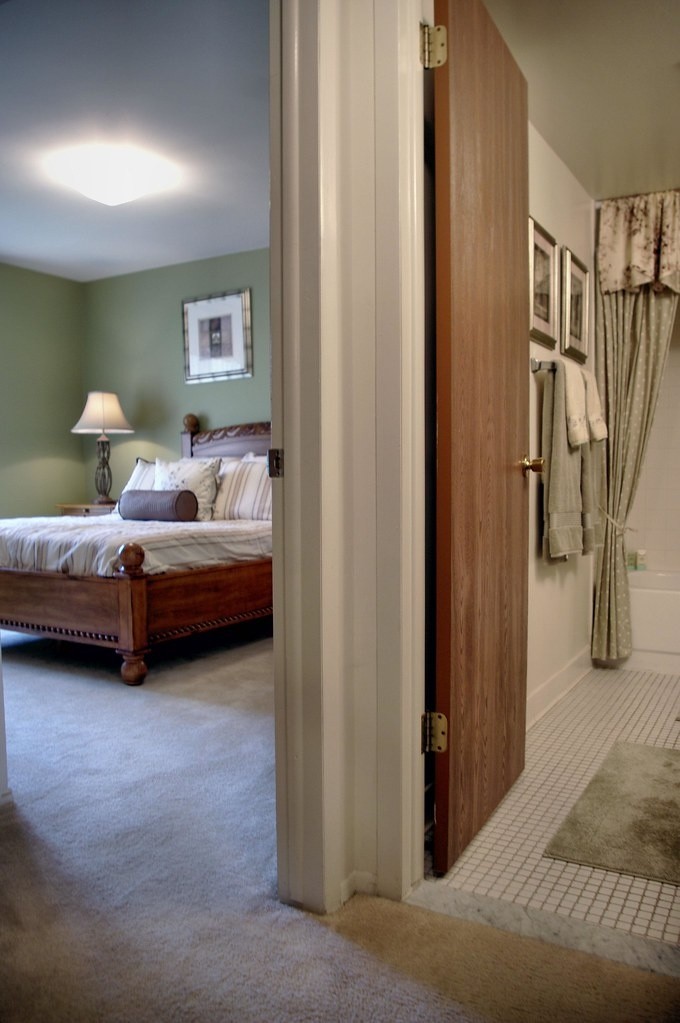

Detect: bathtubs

[626,570,680,656]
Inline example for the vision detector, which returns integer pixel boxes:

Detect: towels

[541,359,608,566]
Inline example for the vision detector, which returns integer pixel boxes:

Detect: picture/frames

[176,285,255,384]
[529,217,557,351]
[559,245,590,364]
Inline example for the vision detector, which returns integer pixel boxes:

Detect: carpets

[544,738,680,887]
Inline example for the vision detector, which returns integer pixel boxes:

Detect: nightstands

[55,502,115,516]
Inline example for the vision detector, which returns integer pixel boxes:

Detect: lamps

[70,391,136,503]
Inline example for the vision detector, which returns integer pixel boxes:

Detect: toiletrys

[626,549,647,571]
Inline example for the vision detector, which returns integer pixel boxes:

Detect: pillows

[105,454,272,523]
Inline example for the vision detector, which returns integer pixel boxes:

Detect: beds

[0,413,272,685]
